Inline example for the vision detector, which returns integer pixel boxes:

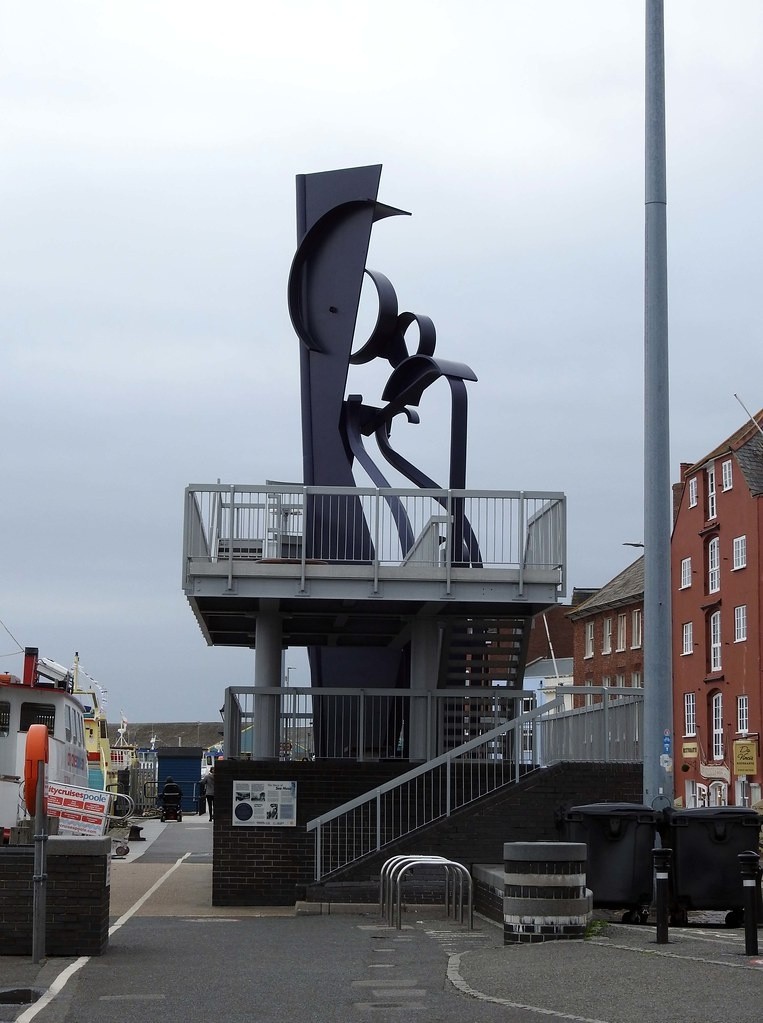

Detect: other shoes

[209,818,213,822]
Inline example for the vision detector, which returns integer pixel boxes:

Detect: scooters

[159,784,182,822]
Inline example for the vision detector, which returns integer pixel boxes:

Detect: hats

[165,776,174,783]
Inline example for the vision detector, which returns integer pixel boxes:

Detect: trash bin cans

[669,804,762,928]
[564,802,658,926]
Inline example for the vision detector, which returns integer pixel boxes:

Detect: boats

[136,733,159,804]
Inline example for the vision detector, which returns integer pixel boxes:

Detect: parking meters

[25,723,51,960]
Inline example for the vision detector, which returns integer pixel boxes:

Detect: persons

[202,767,214,822]
[162,776,183,802]
